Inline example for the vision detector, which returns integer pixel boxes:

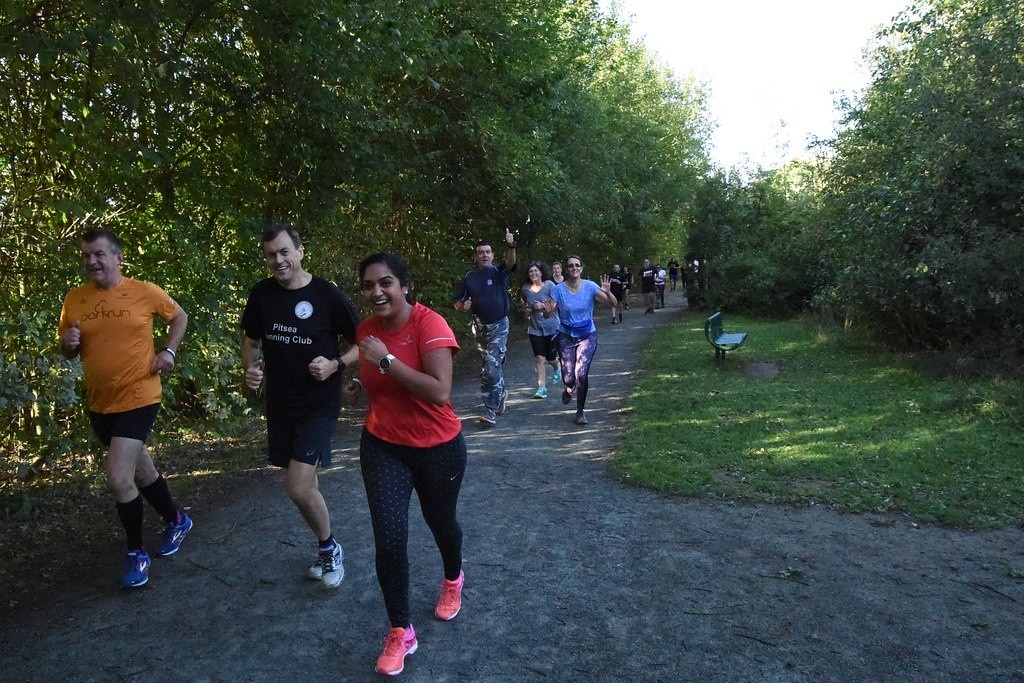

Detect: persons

[653,264,666,309]
[621,266,634,309]
[667,258,679,292]
[57,227,193,589]
[550,263,566,286]
[608,264,624,324]
[453,229,516,425]
[639,259,658,314]
[544,255,619,424]
[239,226,359,588]
[519,260,560,398]
[342,253,467,675]
[681,259,699,290]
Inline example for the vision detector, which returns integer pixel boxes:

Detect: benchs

[704,312,748,364]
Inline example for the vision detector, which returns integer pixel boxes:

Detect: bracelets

[61,340,65,351]
[162,347,175,357]
[353,378,363,389]
[508,241,516,248]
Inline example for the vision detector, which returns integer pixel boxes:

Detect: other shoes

[619,315,622,322]
[612,319,616,322]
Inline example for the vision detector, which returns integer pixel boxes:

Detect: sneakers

[533,386,547,399]
[119,547,150,587]
[577,411,588,424]
[480,409,496,424]
[562,384,576,404]
[158,510,193,556]
[552,363,562,384]
[435,570,464,620]
[497,389,508,414]
[308,552,322,580]
[375,623,418,676]
[319,542,344,588]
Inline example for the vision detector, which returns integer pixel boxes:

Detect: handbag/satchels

[562,319,592,338]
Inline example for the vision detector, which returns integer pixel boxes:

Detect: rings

[313,373,316,375]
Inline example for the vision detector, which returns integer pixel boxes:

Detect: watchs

[335,358,345,370]
[378,353,396,374]
